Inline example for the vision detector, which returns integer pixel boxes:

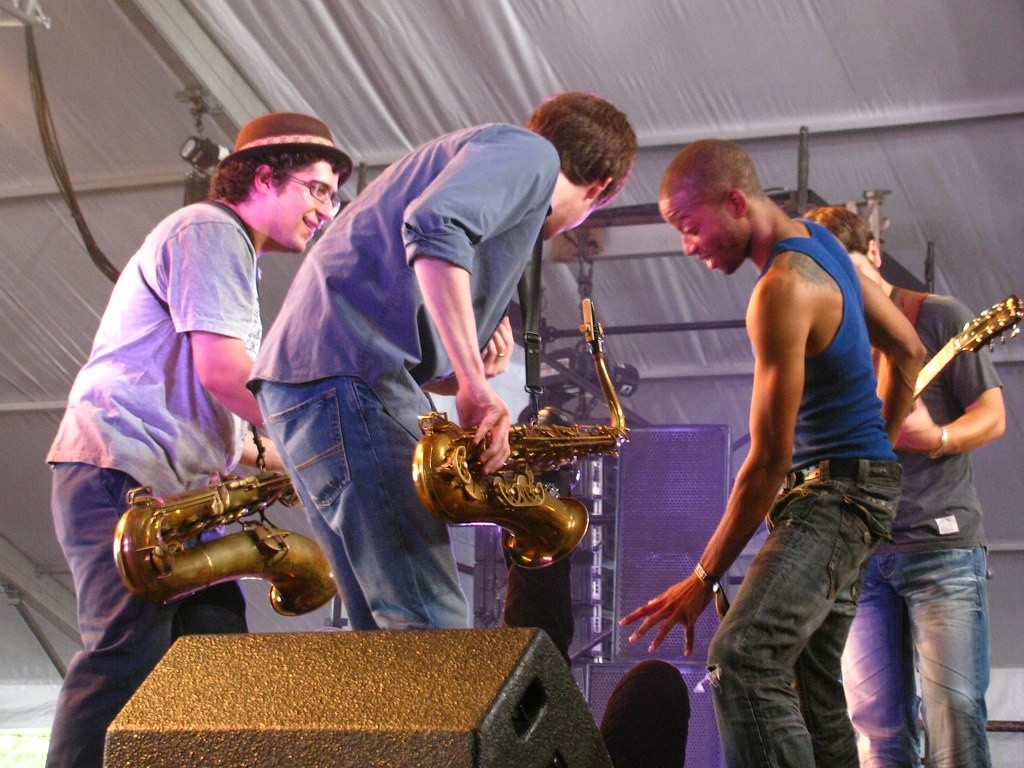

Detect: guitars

[912,291,1023,405]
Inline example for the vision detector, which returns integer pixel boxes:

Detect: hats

[216,112,353,188]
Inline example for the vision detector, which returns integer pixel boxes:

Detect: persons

[45,112,356,768]
[797,207,1007,768]
[618,138,929,768]
[245,91,639,631]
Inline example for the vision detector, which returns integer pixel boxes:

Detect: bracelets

[695,563,730,622]
[930,429,948,459]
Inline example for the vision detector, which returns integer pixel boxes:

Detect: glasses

[272,170,342,217]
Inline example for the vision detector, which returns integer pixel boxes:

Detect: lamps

[181,96,233,176]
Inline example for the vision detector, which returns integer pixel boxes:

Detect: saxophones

[112,467,338,619]
[408,295,632,571]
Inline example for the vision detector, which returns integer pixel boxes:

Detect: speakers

[586,425,728,768]
[103,628,616,768]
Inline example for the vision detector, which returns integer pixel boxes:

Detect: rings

[498,349,507,357]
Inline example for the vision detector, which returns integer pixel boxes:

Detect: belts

[780,456,903,493]
